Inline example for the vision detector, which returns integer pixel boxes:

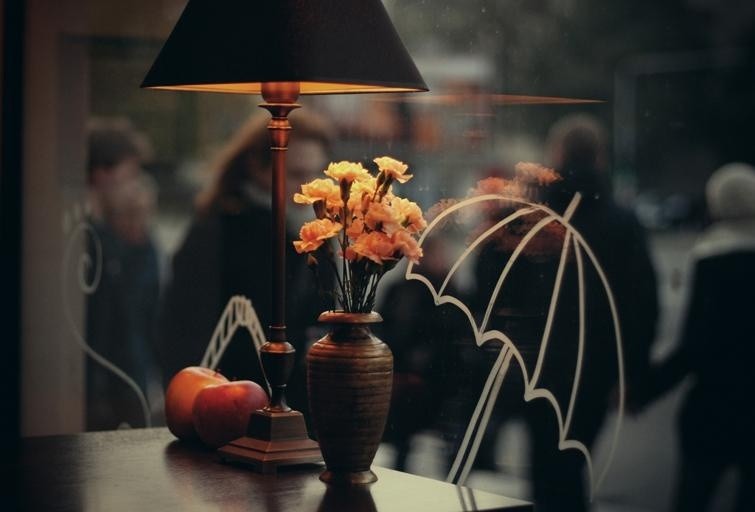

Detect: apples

[190,380,270,463]
[165,367,231,439]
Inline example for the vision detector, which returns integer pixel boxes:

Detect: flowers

[294,147,428,298]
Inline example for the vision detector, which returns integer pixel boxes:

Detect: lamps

[140,1,428,471]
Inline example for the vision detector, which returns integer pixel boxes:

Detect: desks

[2,401,536,512]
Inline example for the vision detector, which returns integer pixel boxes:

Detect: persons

[85,107,351,432]
[370,115,755,505]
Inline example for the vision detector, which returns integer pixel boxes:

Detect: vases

[304,306,395,488]
